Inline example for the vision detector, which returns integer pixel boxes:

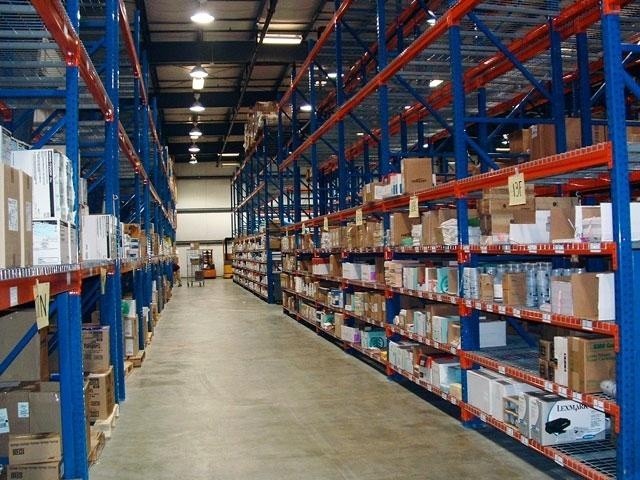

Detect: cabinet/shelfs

[278,0,638,480]
[0,0,176,480]
[231,120,281,305]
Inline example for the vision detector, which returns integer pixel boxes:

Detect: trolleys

[187,253,205,287]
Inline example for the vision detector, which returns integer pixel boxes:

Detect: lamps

[187,66,210,165]
[190,0,216,24]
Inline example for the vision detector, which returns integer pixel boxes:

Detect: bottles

[400,232,414,246]
[481,261,585,309]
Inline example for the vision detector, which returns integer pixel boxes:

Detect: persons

[173,263,182,287]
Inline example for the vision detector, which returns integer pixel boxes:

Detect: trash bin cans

[272,271,283,304]
